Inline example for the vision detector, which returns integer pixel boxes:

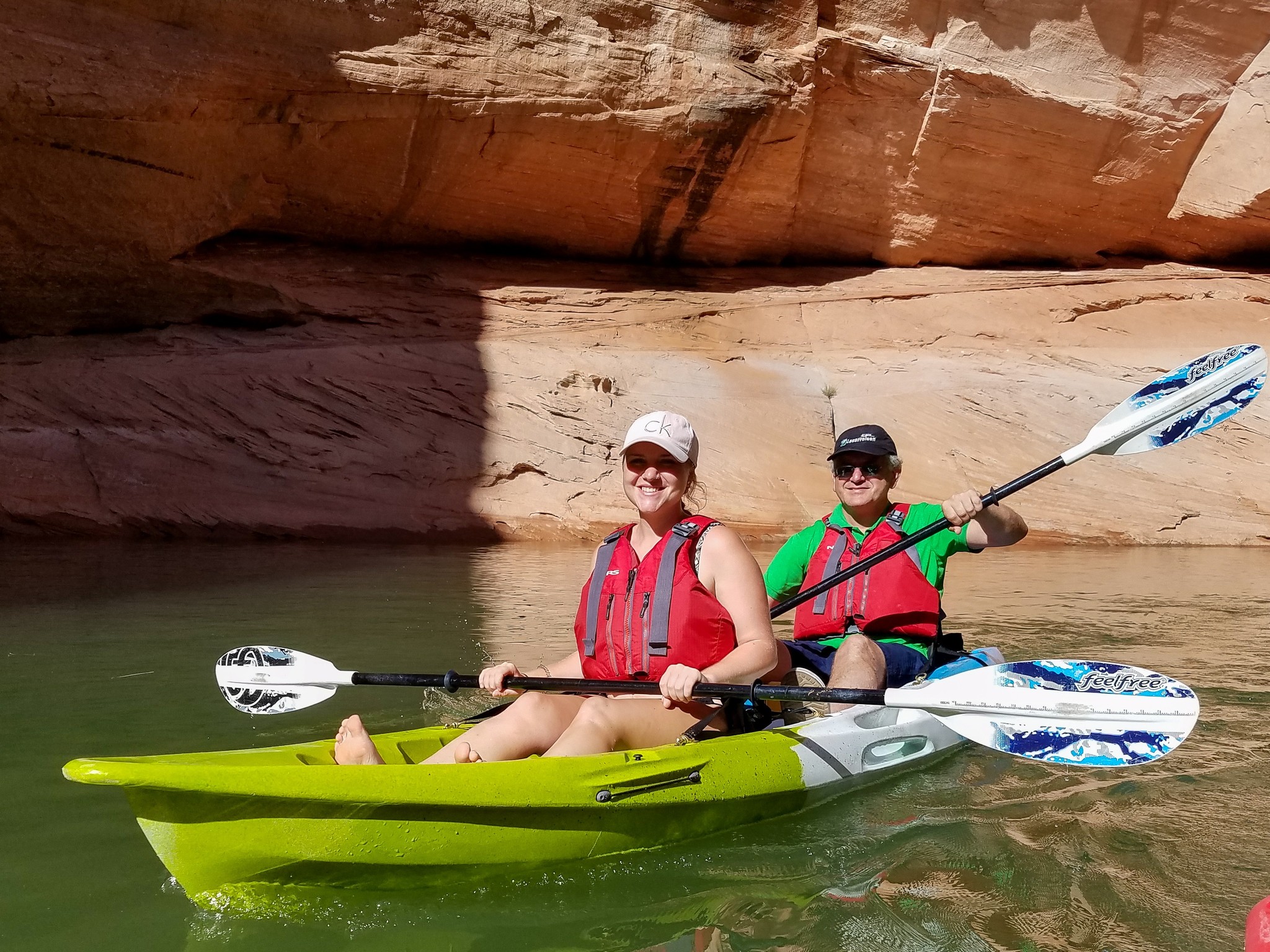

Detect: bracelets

[537,664,551,677]
[521,673,528,693]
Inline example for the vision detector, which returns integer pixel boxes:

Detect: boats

[59,643,1009,909]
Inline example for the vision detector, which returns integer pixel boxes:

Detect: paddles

[210,637,1194,771]
[768,343,1270,603]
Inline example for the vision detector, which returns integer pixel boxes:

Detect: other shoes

[779,667,831,726]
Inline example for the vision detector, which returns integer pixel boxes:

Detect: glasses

[831,461,890,479]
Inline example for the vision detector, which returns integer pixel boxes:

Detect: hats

[619,411,698,470]
[827,425,897,461]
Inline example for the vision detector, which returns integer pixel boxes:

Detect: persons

[335,411,778,765]
[763,424,1028,725]
